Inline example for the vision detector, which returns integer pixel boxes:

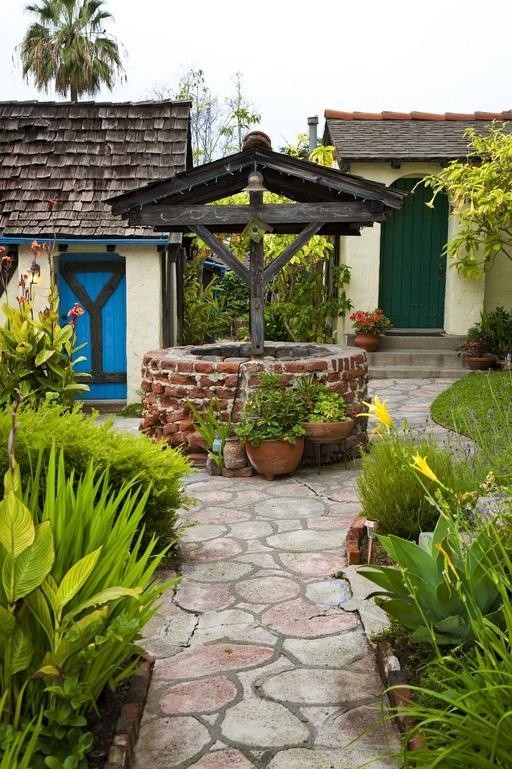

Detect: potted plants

[456,324,497,368]
[183,366,356,479]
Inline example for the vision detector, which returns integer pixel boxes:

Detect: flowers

[350,307,394,335]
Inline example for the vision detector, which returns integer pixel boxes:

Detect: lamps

[241,163,268,192]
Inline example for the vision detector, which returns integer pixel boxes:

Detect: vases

[353,331,380,352]
